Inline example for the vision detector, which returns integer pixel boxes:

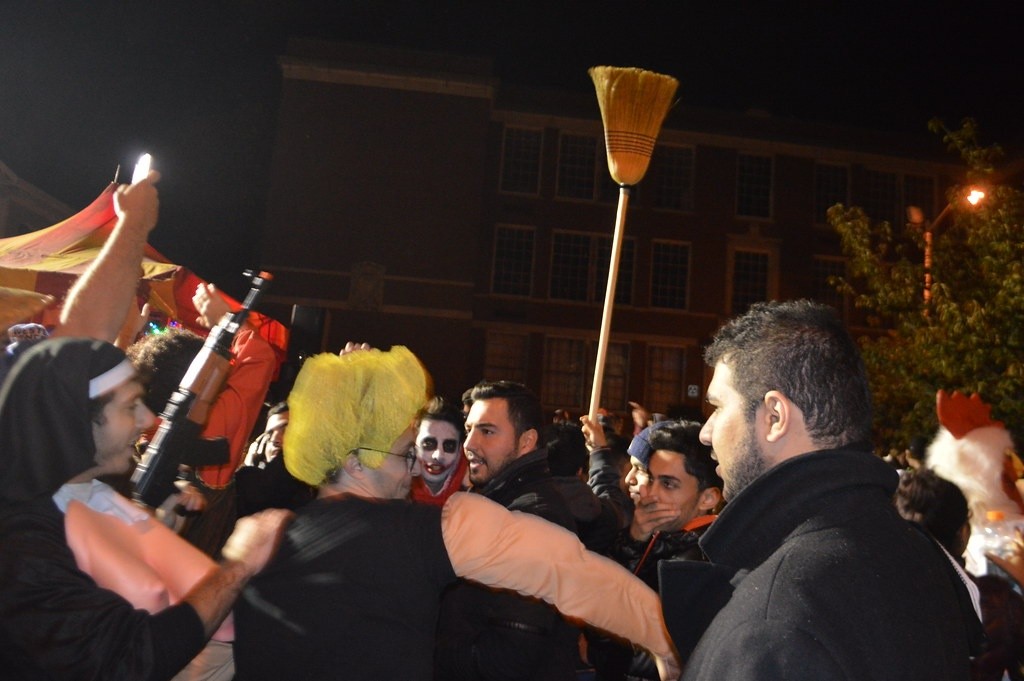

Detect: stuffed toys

[924,388,1024,581]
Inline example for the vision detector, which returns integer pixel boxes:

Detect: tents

[0,170,289,490]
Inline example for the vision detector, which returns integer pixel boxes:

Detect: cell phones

[131,153,153,186]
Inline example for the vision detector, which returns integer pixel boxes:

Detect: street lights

[923,184,984,317]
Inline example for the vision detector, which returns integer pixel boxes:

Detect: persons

[882,442,903,470]
[553,410,568,424]
[236,402,288,471]
[0,337,295,681]
[583,420,723,681]
[655,299,982,681]
[230,344,683,681]
[0,161,159,391]
[896,467,1024,681]
[433,381,579,681]
[406,396,470,505]
[600,414,631,453]
[462,388,473,418]
[544,415,626,554]
[125,282,275,559]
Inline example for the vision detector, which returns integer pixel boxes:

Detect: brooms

[588,64,679,426]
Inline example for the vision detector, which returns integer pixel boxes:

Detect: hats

[627,421,675,466]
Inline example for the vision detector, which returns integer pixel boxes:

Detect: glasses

[348,447,416,471]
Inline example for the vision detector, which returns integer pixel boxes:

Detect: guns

[127,269,269,519]
